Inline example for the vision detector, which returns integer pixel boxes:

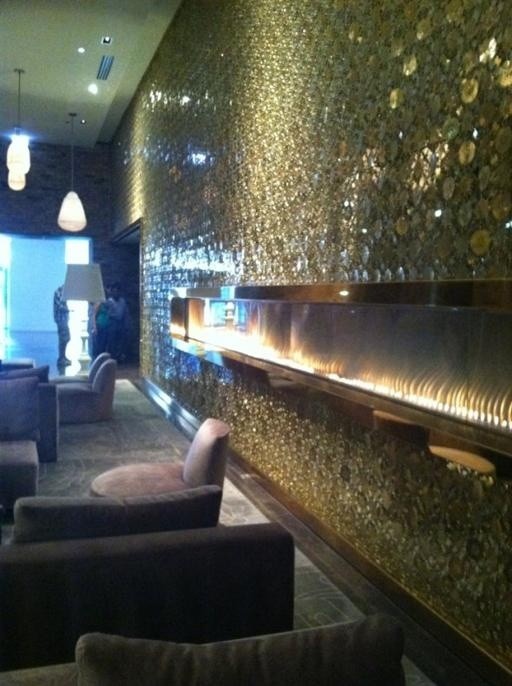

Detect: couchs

[1,350,118,517]
[91,418,231,500]
[3,486,408,686]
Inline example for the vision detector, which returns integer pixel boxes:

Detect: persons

[53,284,72,366]
[87,280,114,366]
[108,281,132,364]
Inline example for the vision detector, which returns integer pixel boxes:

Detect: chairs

[57,356,71,365]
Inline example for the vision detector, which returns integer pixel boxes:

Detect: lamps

[61,262,109,376]
[7,67,32,190]
[57,113,89,233]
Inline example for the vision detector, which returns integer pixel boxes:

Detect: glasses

[7,67,32,190]
[61,262,109,376]
[57,113,89,233]
